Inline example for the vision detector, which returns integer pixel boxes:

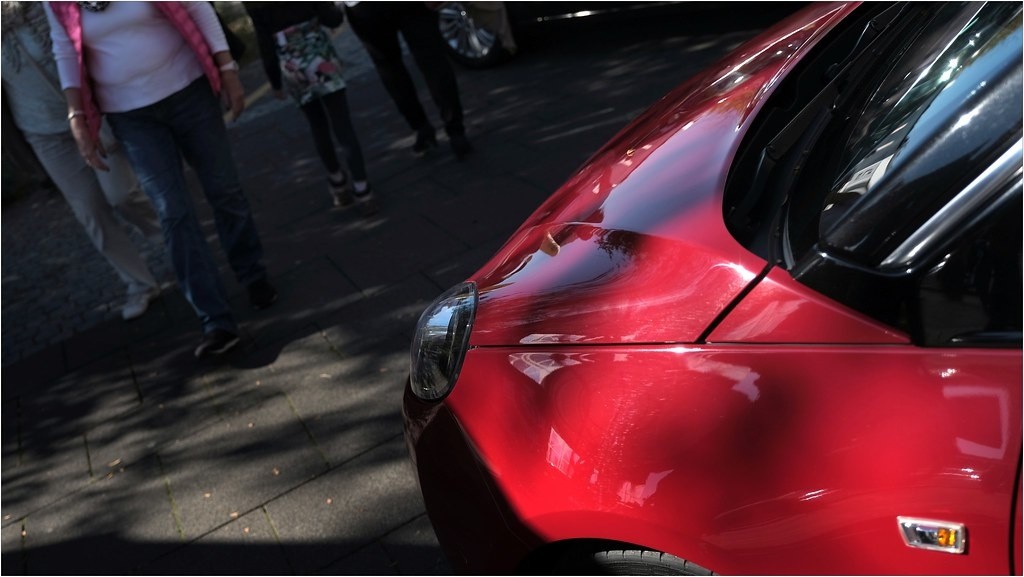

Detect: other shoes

[193,324,242,360]
[121,282,160,319]
[326,165,373,210]
[409,120,468,159]
[249,277,282,309]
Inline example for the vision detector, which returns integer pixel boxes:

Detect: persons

[41,0,275,359]
[345,1,472,158]
[241,1,376,203]
[2,1,162,320]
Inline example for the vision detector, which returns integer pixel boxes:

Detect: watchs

[219,60,239,71]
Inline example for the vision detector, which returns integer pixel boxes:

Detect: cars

[400,1,1022,576]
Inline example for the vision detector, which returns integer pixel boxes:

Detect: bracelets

[66,111,86,121]
